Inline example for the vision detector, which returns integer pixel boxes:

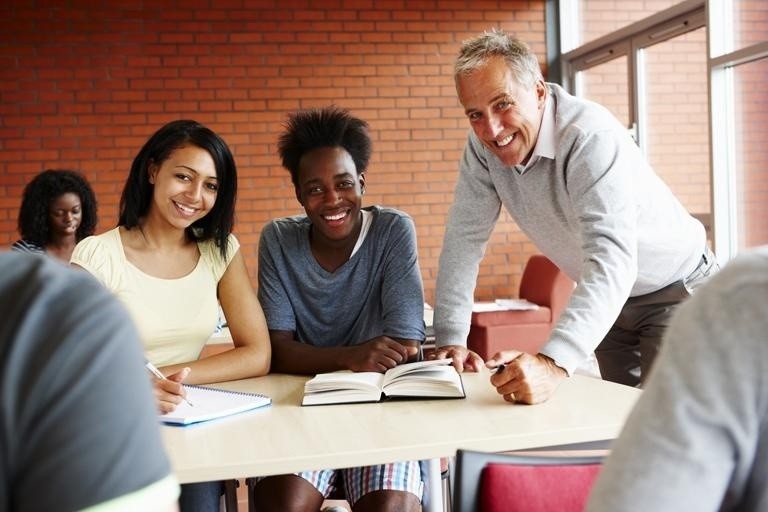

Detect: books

[301,354,466,406]
[161,382,270,427]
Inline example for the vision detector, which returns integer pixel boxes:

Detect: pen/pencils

[147,363,194,407]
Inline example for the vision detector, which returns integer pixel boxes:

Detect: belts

[692,245,708,270]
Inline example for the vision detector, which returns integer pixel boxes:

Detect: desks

[148,369,644,512]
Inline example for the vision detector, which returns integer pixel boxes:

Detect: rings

[509,393,516,403]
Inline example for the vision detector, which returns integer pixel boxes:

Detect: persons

[582,244,766,512]
[0,248,182,512]
[253,102,427,512]
[424,26,719,406]
[66,117,273,512]
[9,167,99,264]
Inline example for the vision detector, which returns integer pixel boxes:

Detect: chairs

[450,449,614,512]
[467,255,575,368]
